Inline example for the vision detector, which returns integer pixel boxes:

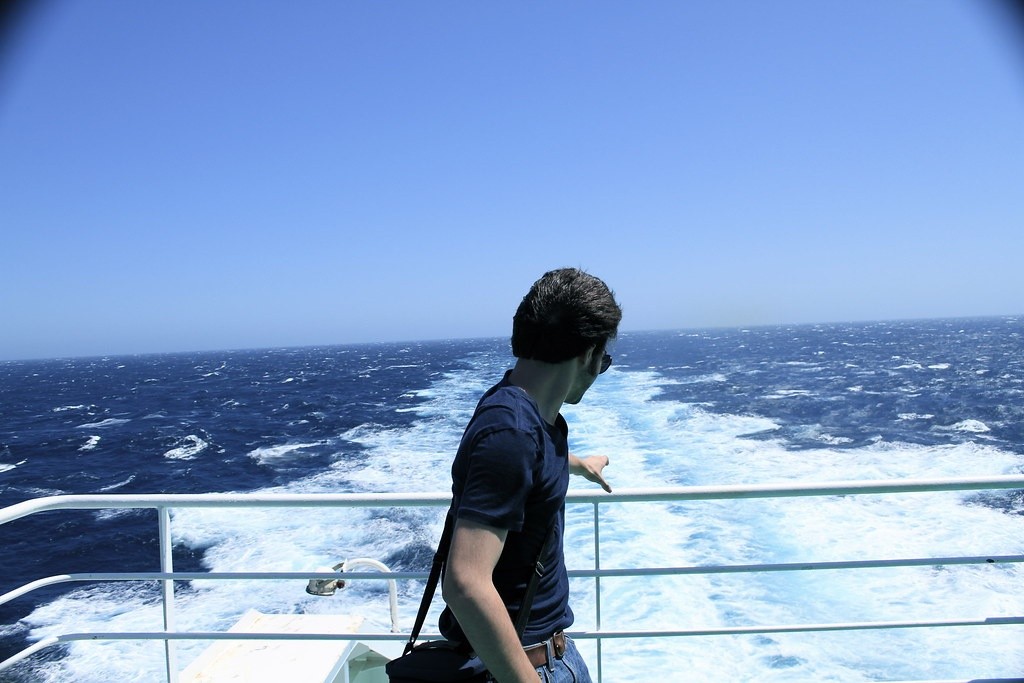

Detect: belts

[524,630,567,670]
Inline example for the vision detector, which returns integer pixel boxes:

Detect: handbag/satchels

[384,641,498,683]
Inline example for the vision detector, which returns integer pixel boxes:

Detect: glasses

[599,343,613,376]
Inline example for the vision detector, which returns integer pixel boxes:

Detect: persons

[442,267,621,683]
